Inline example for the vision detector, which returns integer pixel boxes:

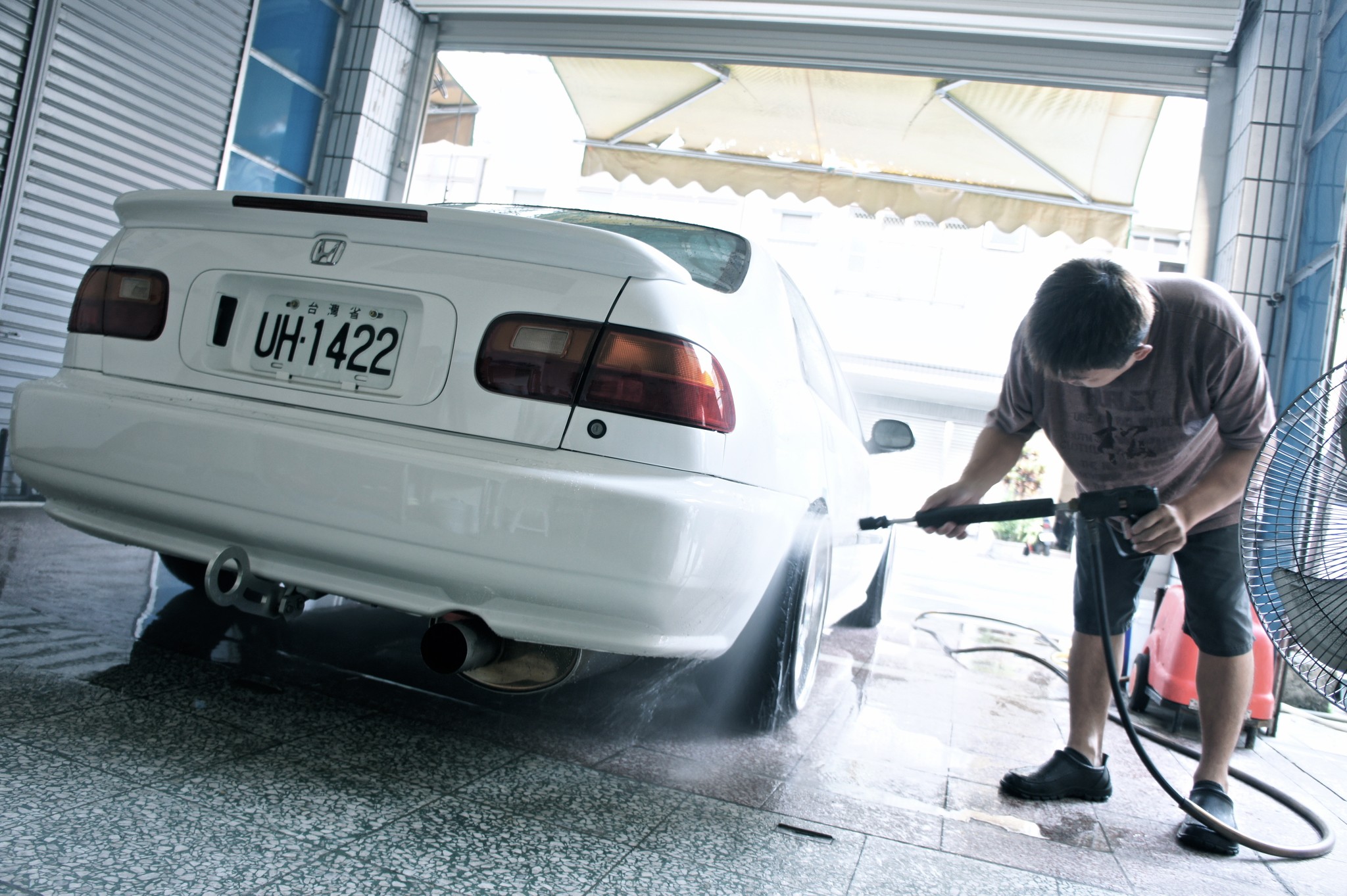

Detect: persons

[916,253,1277,857]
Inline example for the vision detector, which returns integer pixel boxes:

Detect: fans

[1238,360,1346,712]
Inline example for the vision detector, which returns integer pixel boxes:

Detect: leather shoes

[1177,780,1238,853]
[999,747,1112,801]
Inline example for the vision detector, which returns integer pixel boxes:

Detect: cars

[5,188,923,731]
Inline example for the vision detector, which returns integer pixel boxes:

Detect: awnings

[551,55,1166,249]
[422,59,479,146]
